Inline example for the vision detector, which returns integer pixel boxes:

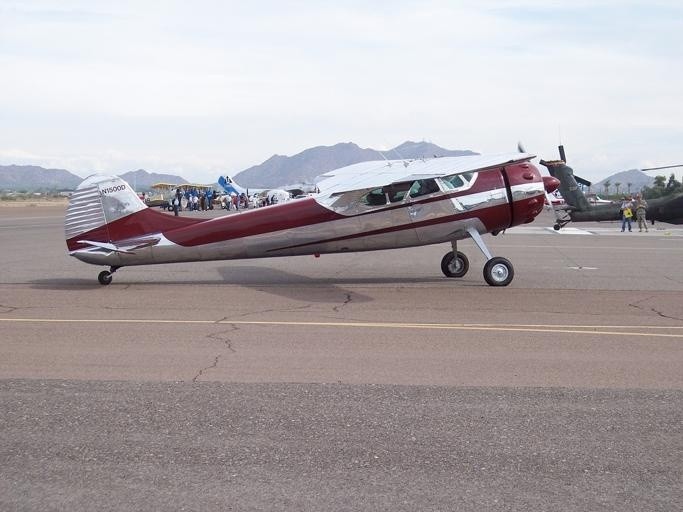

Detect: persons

[619,197,634,232]
[634,193,649,233]
[171,187,275,218]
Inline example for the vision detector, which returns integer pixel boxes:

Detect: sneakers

[639,230,648,232]
[620,230,632,232]
